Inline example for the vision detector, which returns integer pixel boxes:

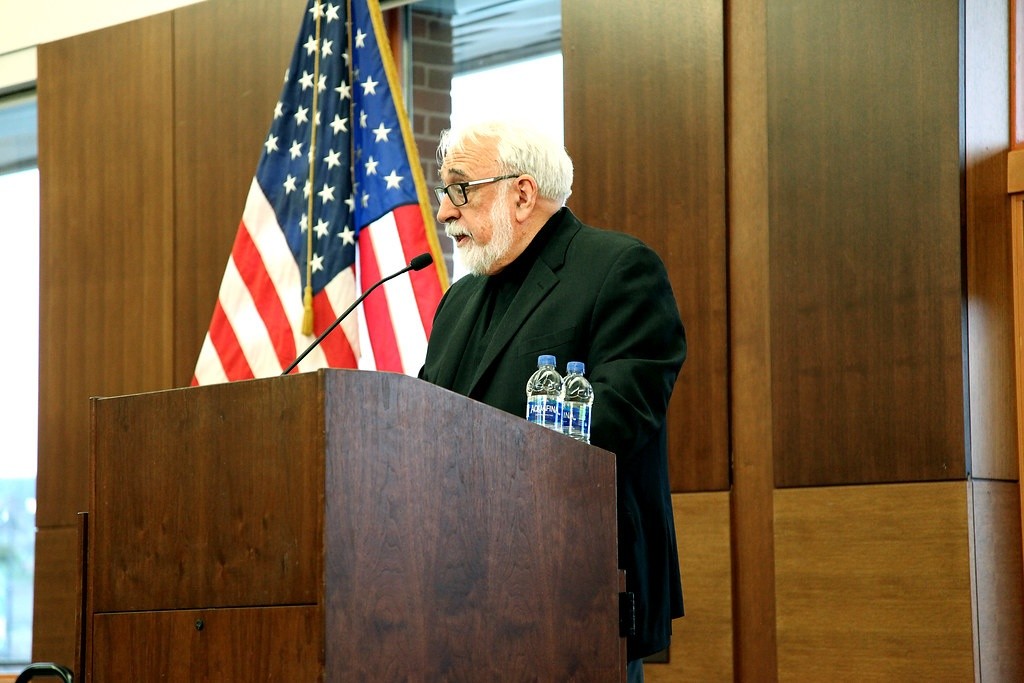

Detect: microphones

[280,253,436,376]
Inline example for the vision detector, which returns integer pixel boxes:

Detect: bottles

[564,361,594,443]
[526,354,565,434]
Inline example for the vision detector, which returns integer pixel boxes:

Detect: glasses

[433,176,520,207]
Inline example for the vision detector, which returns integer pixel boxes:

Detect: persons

[417,113,685,683]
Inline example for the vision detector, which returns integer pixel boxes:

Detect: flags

[188,0,450,387]
